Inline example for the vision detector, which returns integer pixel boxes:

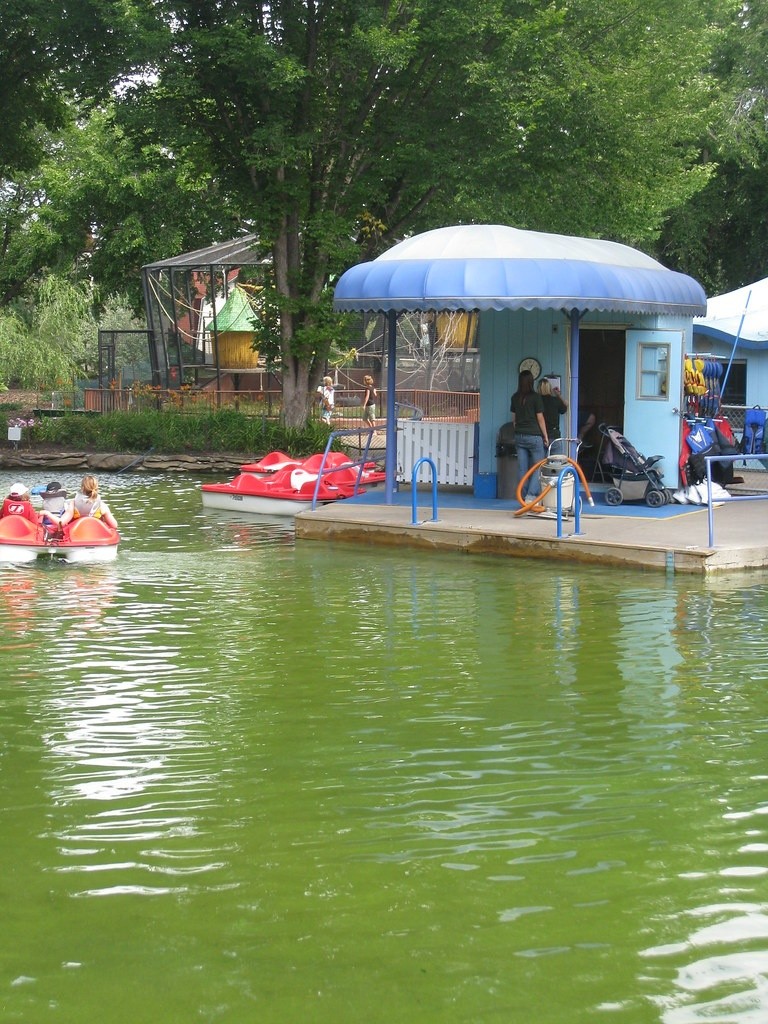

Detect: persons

[317,376,335,426]
[510,370,549,506]
[536,378,567,456]
[40,482,66,524]
[0,483,36,522]
[578,413,609,482]
[38,476,118,529]
[363,375,377,436]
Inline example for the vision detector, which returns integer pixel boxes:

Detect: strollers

[597,422,673,508]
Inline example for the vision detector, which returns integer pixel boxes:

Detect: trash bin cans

[497,423,521,499]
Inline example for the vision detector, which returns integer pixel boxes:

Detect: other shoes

[525,499,539,506]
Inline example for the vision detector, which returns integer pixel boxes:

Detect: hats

[10,483,28,497]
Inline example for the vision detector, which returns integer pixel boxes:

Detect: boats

[239,449,386,486]
[200,463,366,516]
[0,498,122,563]
